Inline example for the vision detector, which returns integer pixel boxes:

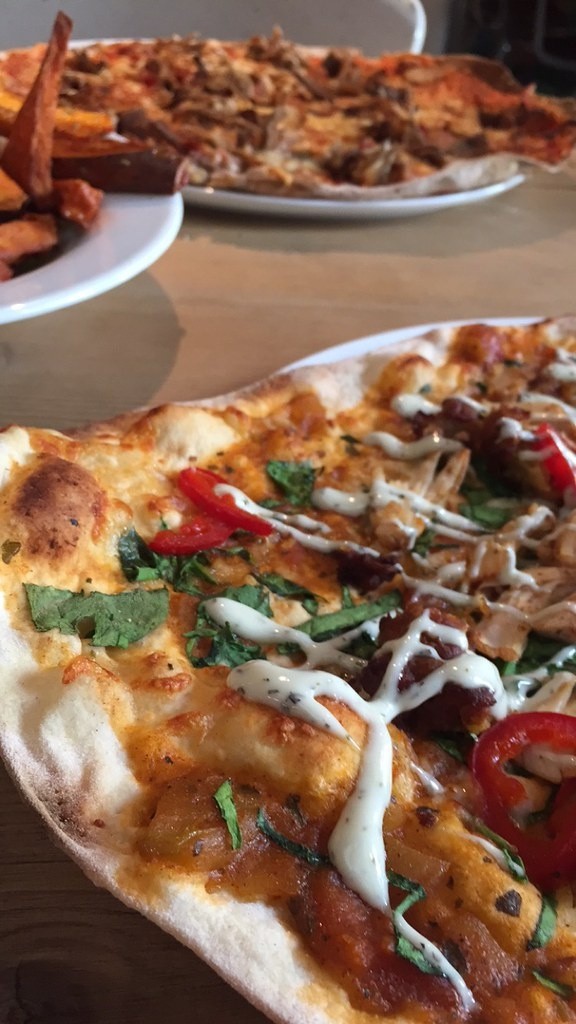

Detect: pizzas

[2,319,575,1024]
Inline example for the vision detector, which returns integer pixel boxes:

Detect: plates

[0,39,525,216]
[274,316,544,371]
[0,125,189,324]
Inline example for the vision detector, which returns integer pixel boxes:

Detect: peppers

[472,708,575,896]
